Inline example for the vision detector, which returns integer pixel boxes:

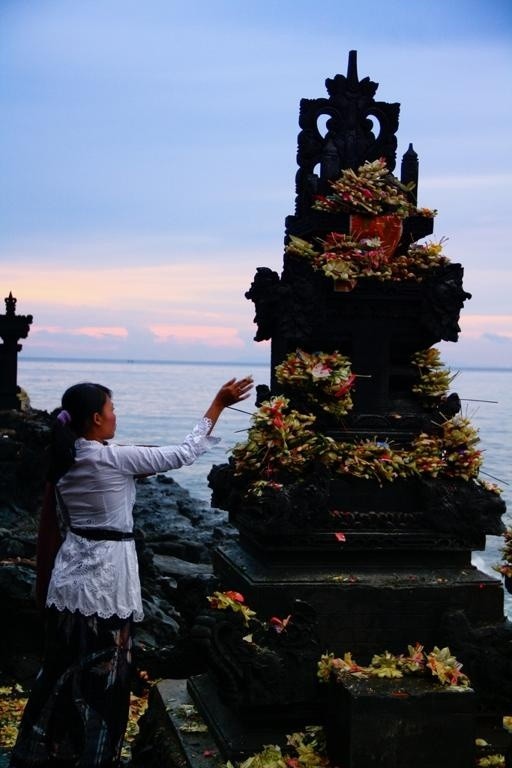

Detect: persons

[45,377,254,768]
[7,408,165,767]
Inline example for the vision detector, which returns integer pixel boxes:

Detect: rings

[237,386,241,392]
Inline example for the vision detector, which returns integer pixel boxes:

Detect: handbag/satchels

[36,482,63,599]
